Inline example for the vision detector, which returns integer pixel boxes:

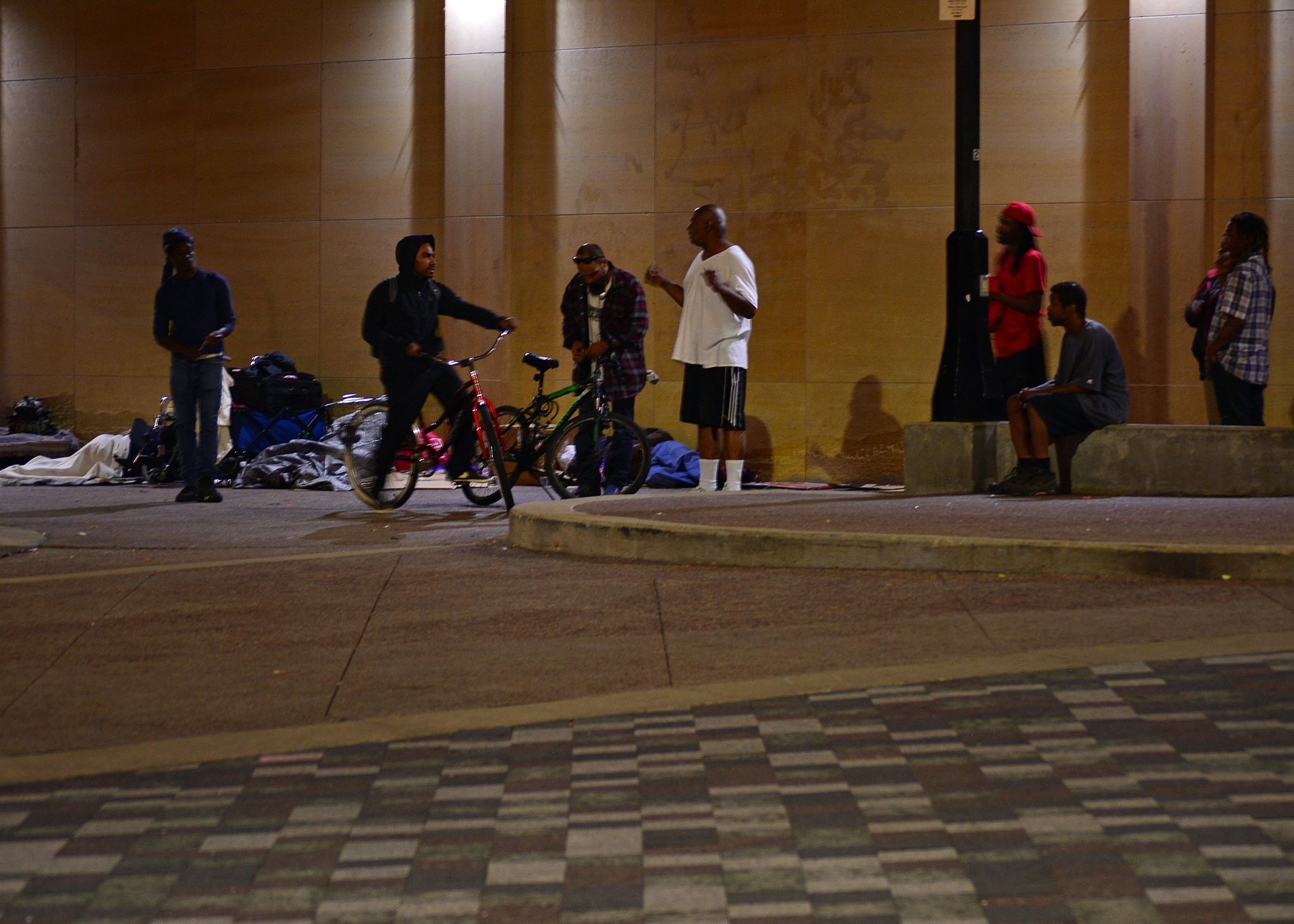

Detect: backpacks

[5,396,53,435]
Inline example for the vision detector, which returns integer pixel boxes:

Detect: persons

[362,233,521,500]
[153,226,237,504]
[989,203,1049,403]
[644,204,758,488]
[986,280,1129,496]
[1185,212,1276,427]
[560,243,649,496]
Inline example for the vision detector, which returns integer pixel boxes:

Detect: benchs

[995,420,1294,499]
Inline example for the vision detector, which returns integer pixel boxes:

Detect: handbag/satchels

[262,371,321,416]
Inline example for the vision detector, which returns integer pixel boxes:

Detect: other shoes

[370,466,388,492]
[174,483,196,502]
[602,485,619,495]
[445,467,495,483]
[196,487,223,503]
[561,486,603,499]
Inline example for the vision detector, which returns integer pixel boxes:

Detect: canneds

[543,476,550,486]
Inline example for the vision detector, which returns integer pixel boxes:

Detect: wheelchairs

[112,353,251,489]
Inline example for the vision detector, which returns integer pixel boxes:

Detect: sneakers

[988,467,1031,494]
[1006,469,1057,497]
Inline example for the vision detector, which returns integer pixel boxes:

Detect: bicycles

[337,325,515,513]
[459,351,653,506]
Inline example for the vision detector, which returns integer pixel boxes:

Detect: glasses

[572,255,604,265]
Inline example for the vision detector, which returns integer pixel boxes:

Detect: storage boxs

[494,424,566,487]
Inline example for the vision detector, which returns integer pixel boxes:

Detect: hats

[161,226,194,255]
[1000,200,1045,238]
